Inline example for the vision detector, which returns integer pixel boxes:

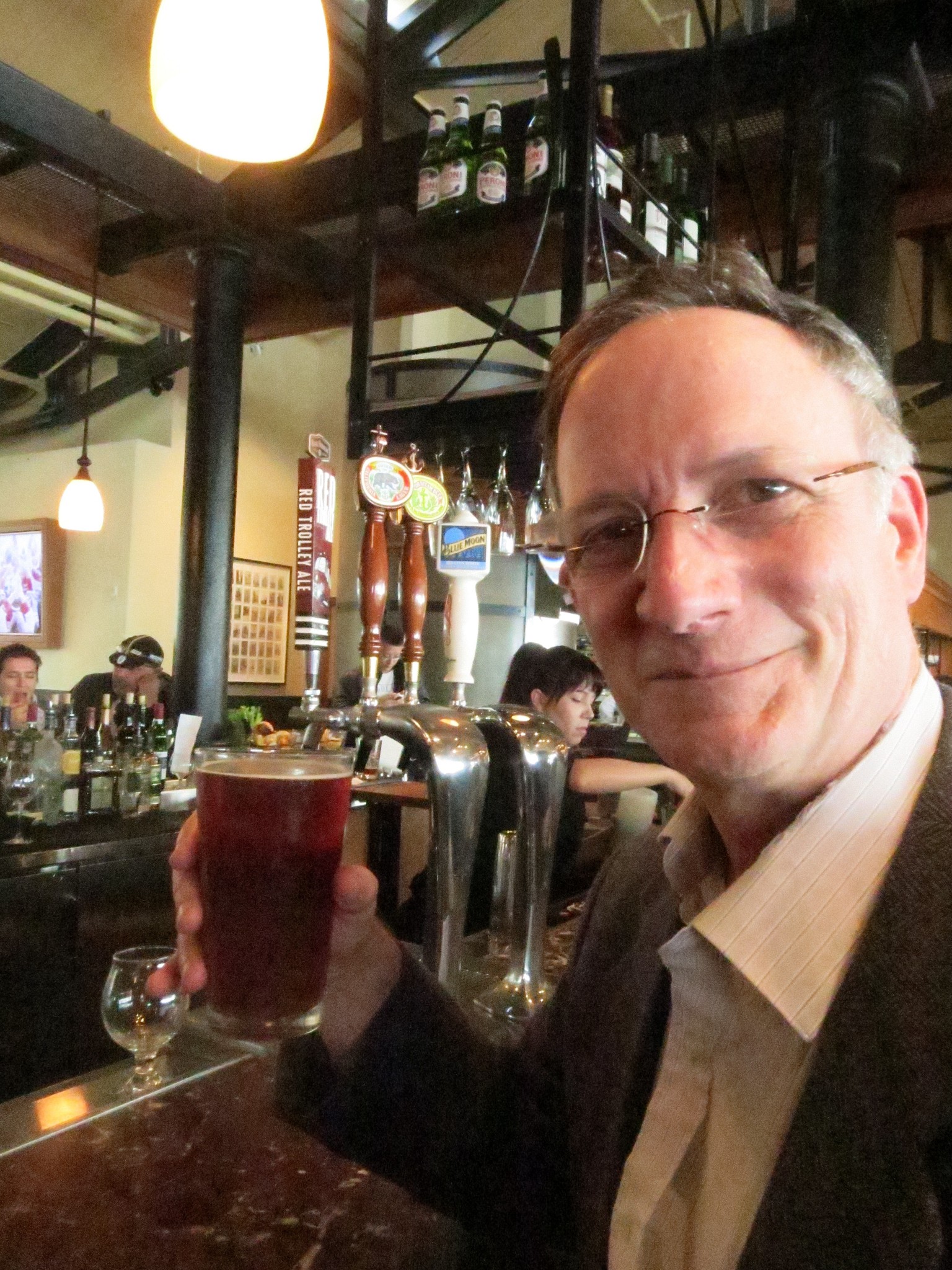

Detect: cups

[189,741,358,1039]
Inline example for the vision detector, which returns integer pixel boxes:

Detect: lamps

[57,178,106,532]
[149,0,332,164]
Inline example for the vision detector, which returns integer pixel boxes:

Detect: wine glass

[437,441,560,553]
[102,943,188,1102]
[3,758,36,845]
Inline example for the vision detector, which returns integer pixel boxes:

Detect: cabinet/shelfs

[348,0,732,494]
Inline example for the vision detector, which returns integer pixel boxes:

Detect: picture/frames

[227,557,293,685]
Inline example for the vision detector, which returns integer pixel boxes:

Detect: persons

[63,635,173,755]
[387,642,695,938]
[146,246,952,1270]
[0,645,45,732]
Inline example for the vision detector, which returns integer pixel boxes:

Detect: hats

[109,634,163,668]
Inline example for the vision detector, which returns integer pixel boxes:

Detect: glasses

[515,461,882,592]
[381,654,401,662]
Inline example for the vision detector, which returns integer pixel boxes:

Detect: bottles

[523,71,567,193]
[474,102,512,208]
[418,106,446,217]
[0,691,172,829]
[448,92,478,215]
[590,83,704,273]
